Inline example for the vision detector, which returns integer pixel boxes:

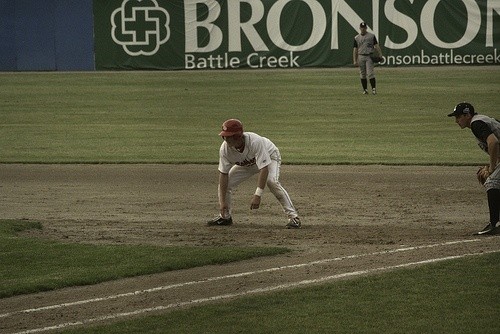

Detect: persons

[207,119,302,229]
[447,102,500,235]
[353,22,381,95]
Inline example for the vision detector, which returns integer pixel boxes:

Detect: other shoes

[471,222,500,236]
[372,88,376,95]
[362,89,369,95]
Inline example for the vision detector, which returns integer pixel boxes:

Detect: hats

[217,119,244,137]
[359,22,366,28]
[447,101,474,117]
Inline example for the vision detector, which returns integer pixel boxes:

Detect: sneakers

[285,217,301,229]
[207,216,233,227]
